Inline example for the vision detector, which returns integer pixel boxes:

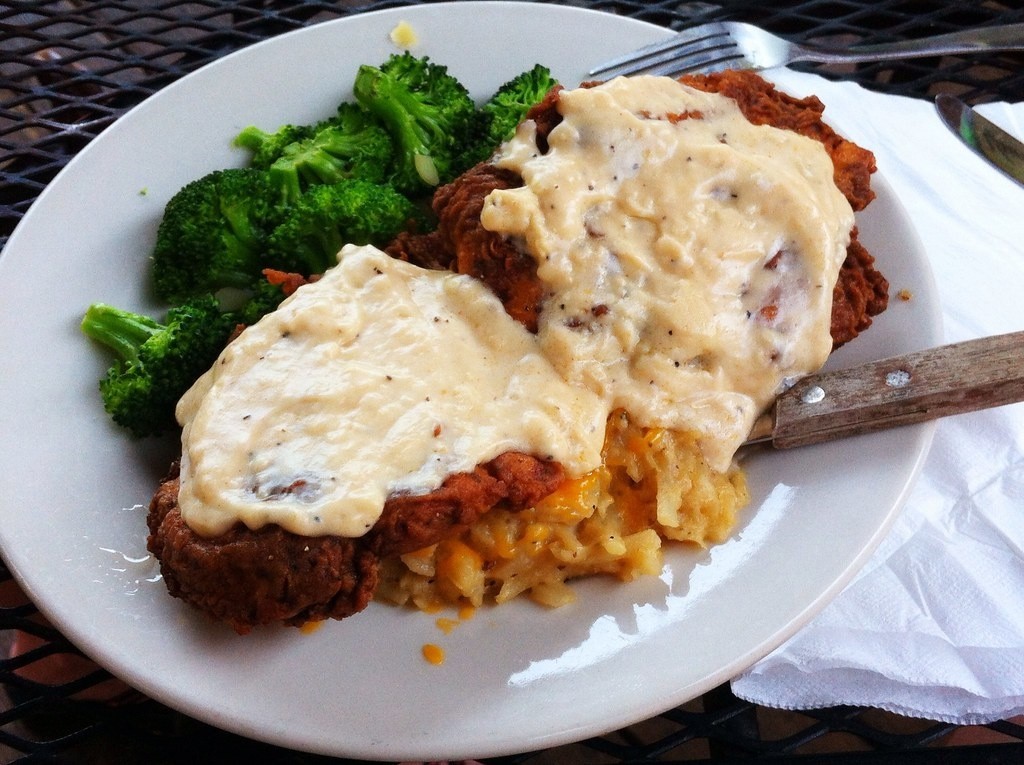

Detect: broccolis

[79,48,561,440]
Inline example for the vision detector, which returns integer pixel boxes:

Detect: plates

[0,0,944,759]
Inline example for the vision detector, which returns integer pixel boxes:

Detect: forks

[588,21,1024,82]
